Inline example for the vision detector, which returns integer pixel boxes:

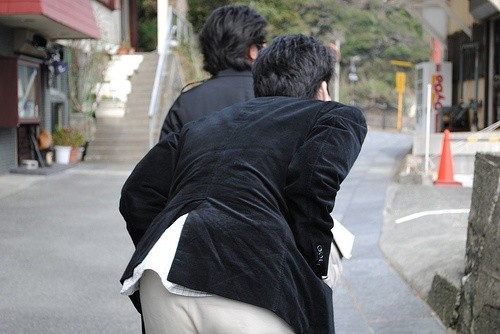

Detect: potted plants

[119,41,136,55]
[53,124,85,165]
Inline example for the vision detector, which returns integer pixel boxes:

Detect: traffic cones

[432,128,463,186]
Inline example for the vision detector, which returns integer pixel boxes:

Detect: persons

[120,31,367,334]
[159,5,272,144]
